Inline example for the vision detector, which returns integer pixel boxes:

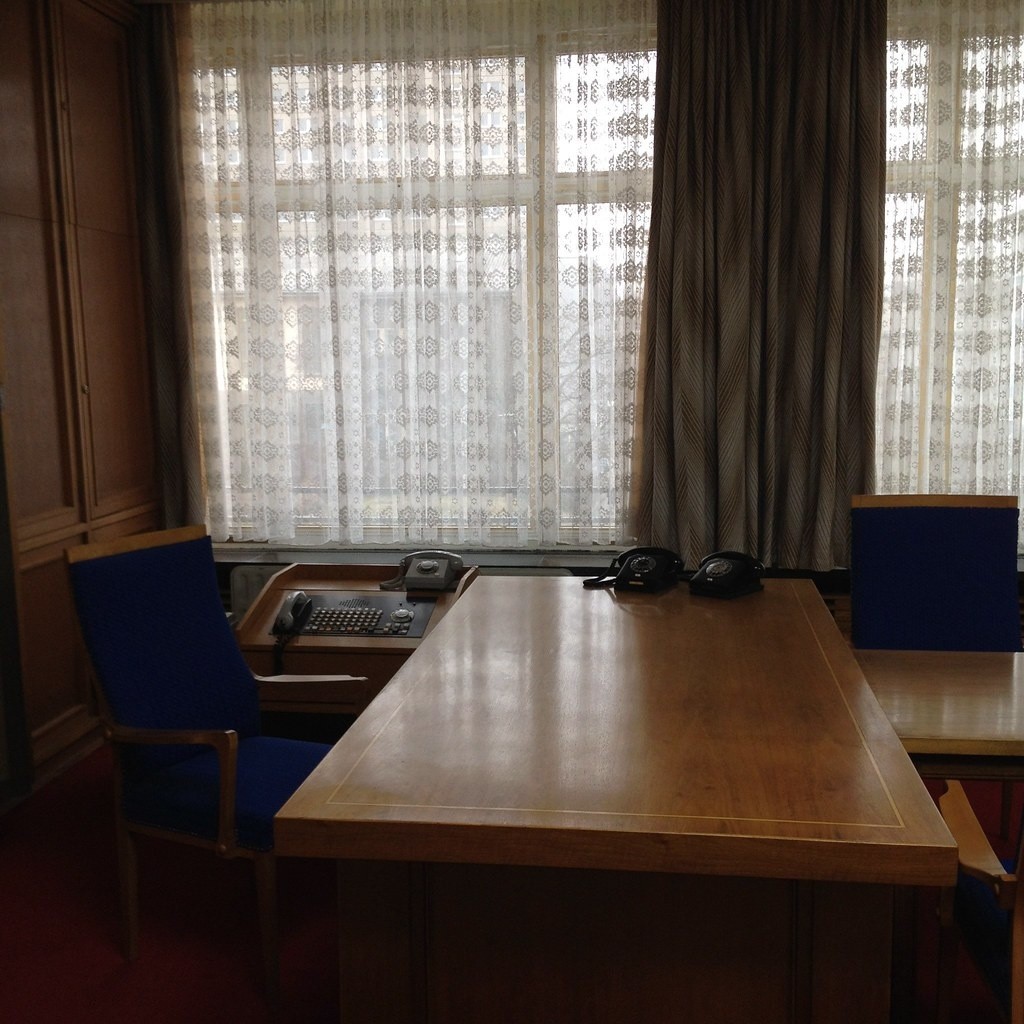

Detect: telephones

[690,552,765,599]
[275,590,312,639]
[400,549,463,590]
[613,546,684,593]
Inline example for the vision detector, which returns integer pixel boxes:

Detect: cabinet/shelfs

[0,0,156,793]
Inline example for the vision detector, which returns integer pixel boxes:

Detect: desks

[855,646,1024,841]
[272,574,961,1024]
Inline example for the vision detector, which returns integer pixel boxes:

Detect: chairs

[63,523,371,1015]
[849,495,1020,652]
[937,779,1024,1024]
